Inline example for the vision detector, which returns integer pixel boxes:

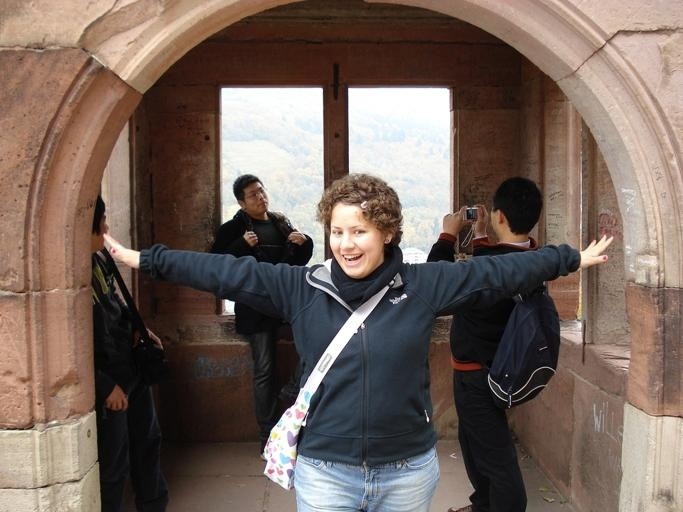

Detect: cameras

[463,207,480,221]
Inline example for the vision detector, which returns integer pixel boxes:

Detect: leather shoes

[448,504,475,512]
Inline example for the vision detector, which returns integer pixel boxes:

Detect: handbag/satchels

[261,401,308,492]
[135,342,175,387]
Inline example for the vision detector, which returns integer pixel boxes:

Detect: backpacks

[484,288,561,409]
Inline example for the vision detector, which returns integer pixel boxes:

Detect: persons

[90,196,168,512]
[103,174,615,512]
[208,175,313,461]
[427,177,543,512]
